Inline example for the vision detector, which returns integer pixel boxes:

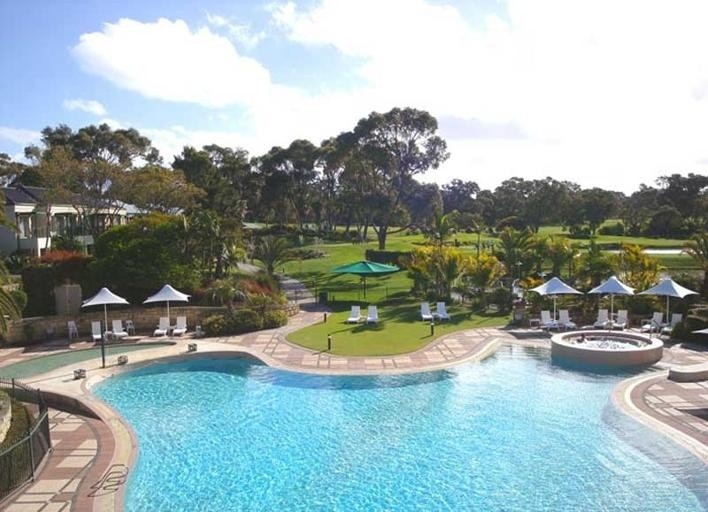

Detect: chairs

[420,302,451,321]
[90,320,136,343]
[347,305,378,325]
[153,316,188,338]
[538,308,682,339]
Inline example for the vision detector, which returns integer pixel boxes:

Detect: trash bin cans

[320,292,328,303]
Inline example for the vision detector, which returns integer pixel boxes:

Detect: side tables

[529,319,540,326]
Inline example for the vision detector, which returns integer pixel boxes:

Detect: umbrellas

[527,277,585,321]
[143,284,192,325]
[587,276,637,320]
[80,287,129,331]
[636,278,701,323]
[331,261,400,298]
